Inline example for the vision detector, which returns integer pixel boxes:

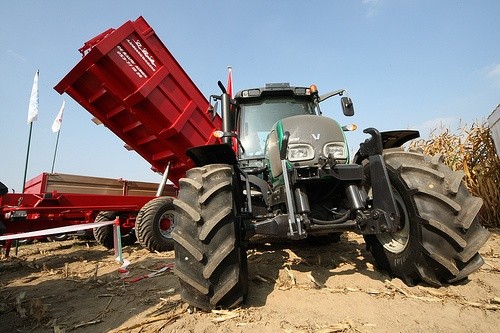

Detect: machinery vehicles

[171,81,492,312]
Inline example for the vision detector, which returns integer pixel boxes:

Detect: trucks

[0,16,238,254]
[24,171,174,245]
